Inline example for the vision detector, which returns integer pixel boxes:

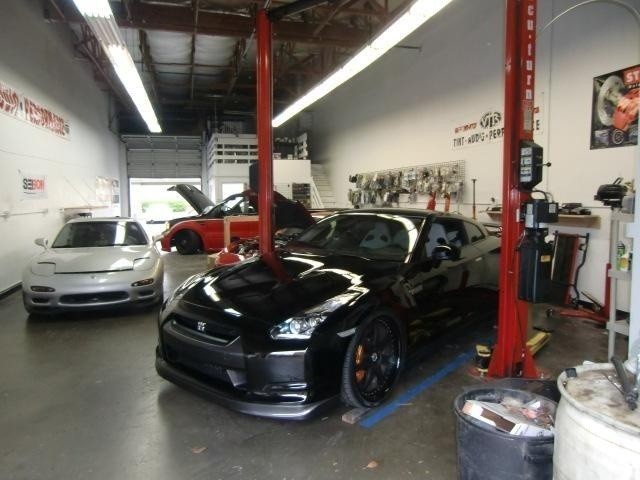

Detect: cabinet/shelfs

[606,211,633,363]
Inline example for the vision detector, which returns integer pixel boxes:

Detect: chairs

[73,228,96,247]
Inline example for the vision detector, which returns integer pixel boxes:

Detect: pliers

[611,356,640,411]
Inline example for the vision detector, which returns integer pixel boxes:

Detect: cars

[161,183,317,254]
[155,209,502,420]
[23,216,164,315]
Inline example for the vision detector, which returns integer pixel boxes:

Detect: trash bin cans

[452,362,639,479]
[213,253,242,266]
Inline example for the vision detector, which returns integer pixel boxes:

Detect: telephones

[533,199,561,223]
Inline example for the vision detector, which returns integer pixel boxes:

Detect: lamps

[72,0,165,133]
[271,0,453,127]
